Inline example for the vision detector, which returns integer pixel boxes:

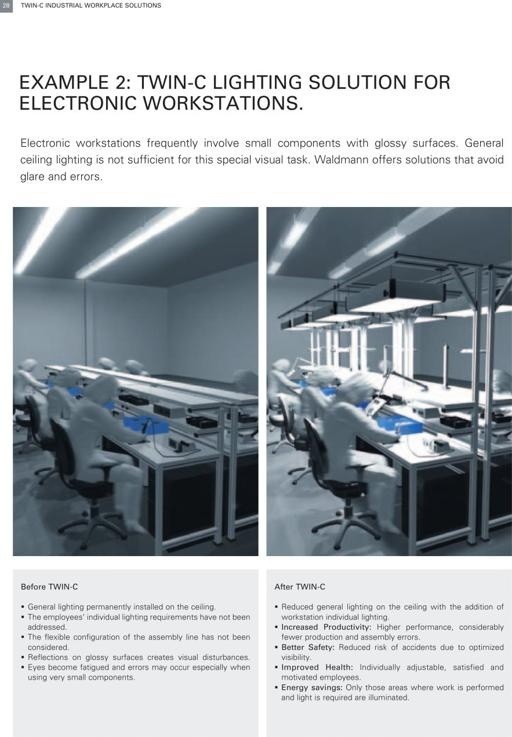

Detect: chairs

[13,394,127,550]
[267,391,381,550]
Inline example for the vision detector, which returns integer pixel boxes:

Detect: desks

[34,364,258,555]
[285,378,512,556]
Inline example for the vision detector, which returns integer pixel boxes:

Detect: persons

[60,373,152,536]
[97,355,120,372]
[39,367,102,451]
[317,370,403,537]
[13,357,50,412]
[126,358,150,378]
[293,366,333,445]
[267,356,302,409]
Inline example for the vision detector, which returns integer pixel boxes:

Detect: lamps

[13,207,197,280]
[266,207,512,331]
